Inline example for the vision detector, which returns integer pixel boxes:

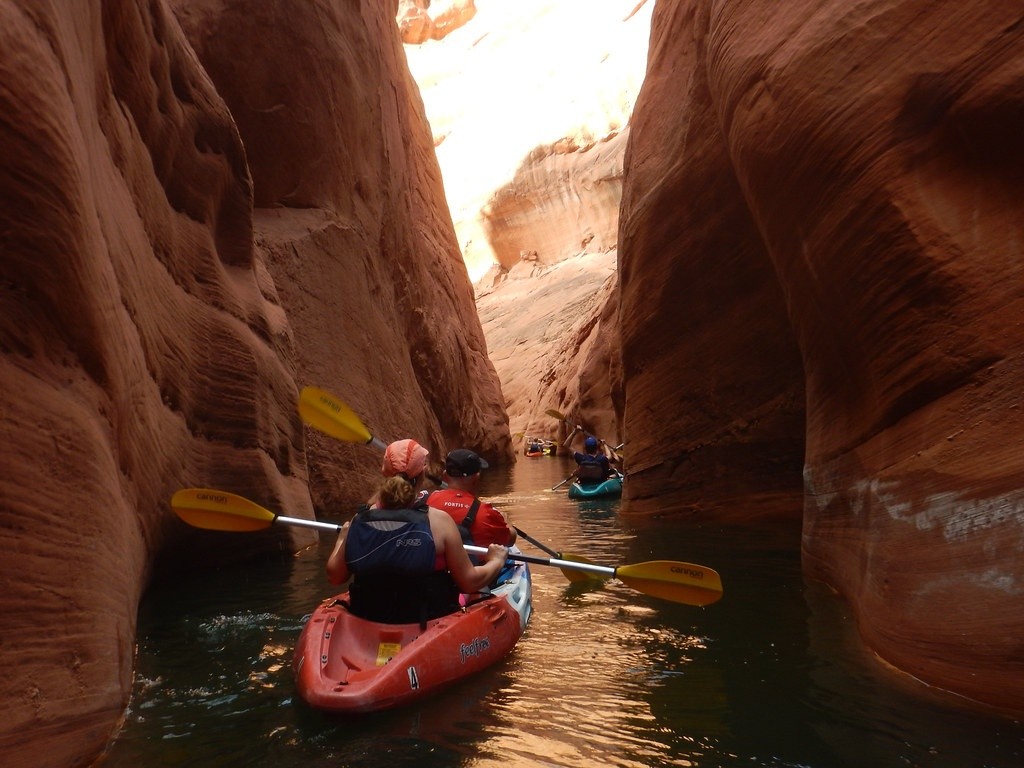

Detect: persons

[527,437,545,453]
[325,438,518,625]
[563,425,620,484]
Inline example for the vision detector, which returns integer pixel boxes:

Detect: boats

[568,478,624,501]
[525,452,544,458]
[291,544,533,715]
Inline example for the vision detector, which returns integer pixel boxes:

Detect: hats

[585,437,596,452]
[446,449,488,477]
[534,437,538,442]
[382,438,429,478]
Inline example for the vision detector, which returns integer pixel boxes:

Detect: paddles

[550,439,625,492]
[514,428,558,454]
[294,377,608,582]
[545,407,625,460]
[170,488,722,610]
